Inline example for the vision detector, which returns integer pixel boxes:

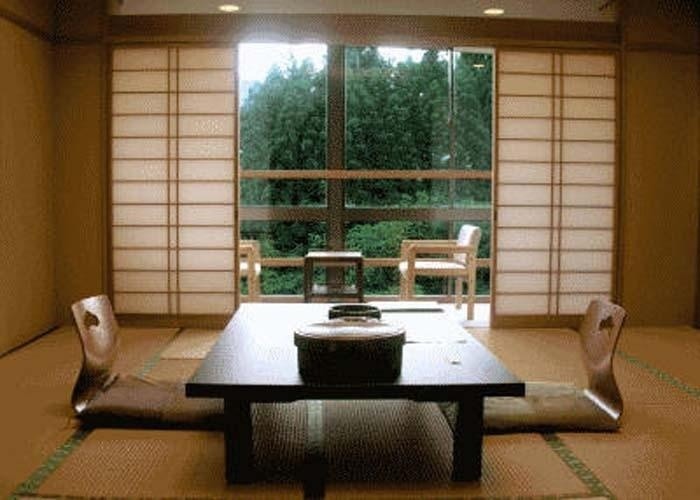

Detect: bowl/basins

[329,304,380,321]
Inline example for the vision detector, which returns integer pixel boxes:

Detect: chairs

[239,240,262,303]
[483,297,627,436]
[398,225,482,320]
[67,293,227,425]
